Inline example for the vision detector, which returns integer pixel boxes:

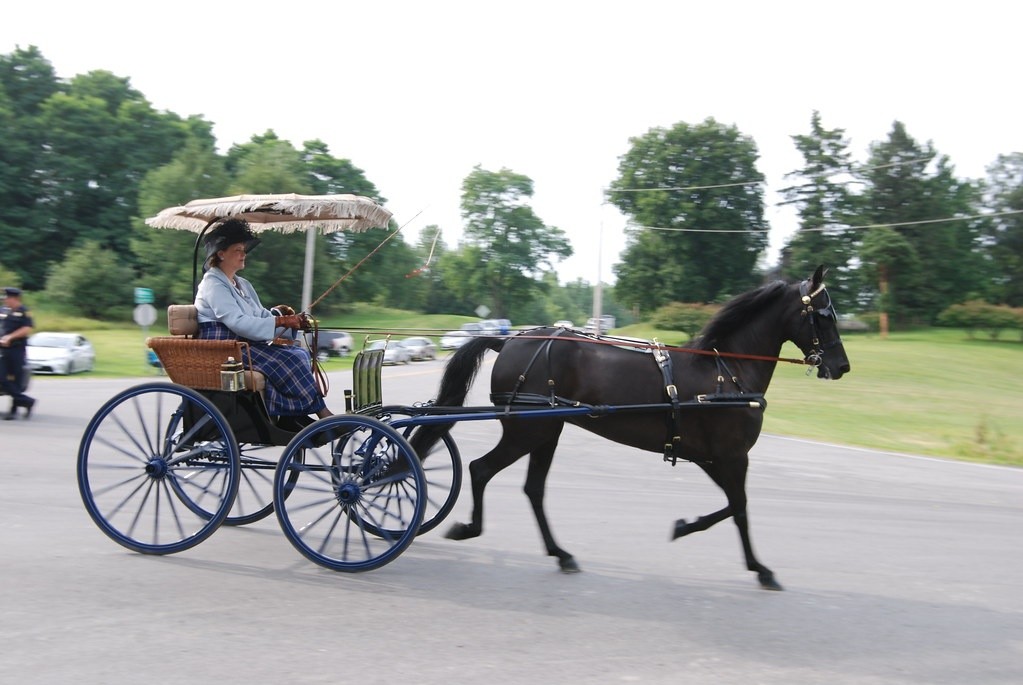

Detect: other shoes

[26,399,34,417]
[0,411,16,420]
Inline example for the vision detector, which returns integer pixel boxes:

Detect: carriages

[75,192,850,593]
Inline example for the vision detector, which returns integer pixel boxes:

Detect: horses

[378,265,851,590]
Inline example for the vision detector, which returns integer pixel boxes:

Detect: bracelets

[277,316,289,329]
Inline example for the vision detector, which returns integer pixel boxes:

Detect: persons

[193,219,333,424]
[0,288,35,421]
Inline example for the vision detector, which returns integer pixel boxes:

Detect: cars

[275,313,616,365]
[24,331,98,376]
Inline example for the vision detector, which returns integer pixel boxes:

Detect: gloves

[277,311,310,330]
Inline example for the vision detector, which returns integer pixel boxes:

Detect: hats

[0,288,21,297]
[202,218,261,275]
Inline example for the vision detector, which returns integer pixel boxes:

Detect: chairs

[168,304,265,393]
